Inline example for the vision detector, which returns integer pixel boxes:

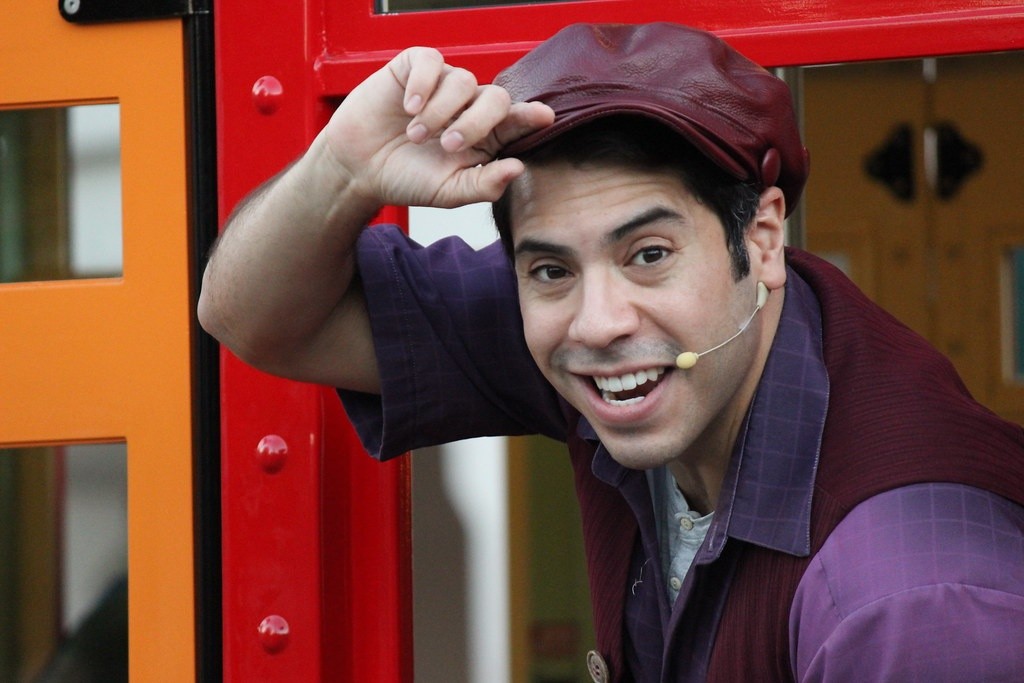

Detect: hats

[490,22,810,221]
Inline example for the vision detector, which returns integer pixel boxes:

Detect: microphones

[676,280,771,370]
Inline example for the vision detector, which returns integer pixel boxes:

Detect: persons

[197,21,1024,683]
